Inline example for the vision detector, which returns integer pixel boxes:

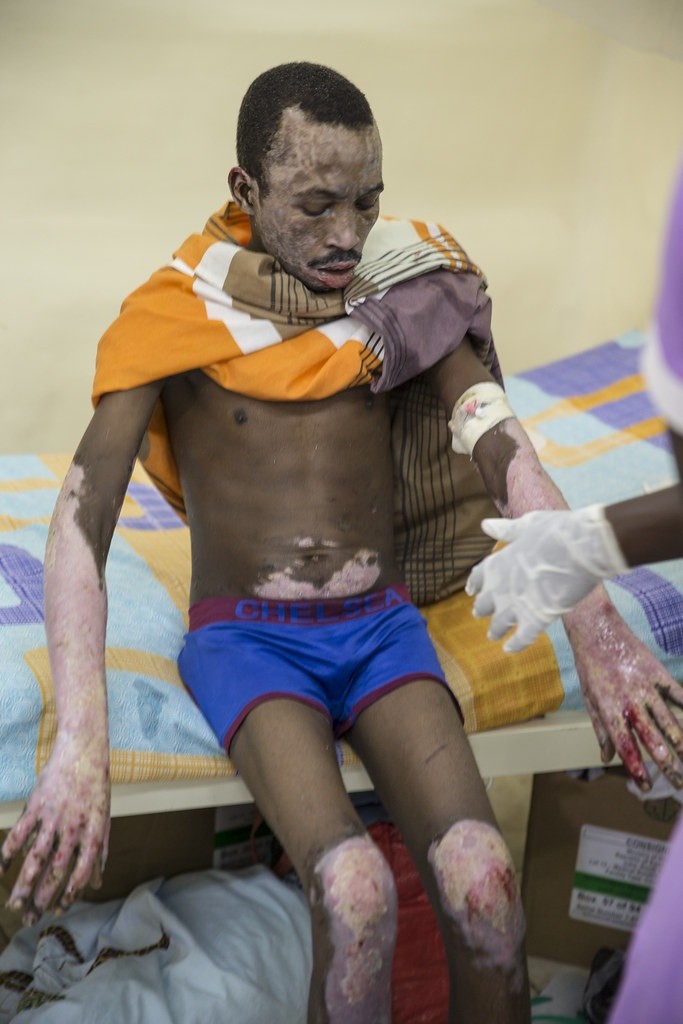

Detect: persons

[0,60,683,1024]
[465,136,683,1024]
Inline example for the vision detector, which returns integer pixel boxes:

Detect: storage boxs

[517,759,682,967]
[62,805,287,902]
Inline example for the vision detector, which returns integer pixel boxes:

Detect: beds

[0,327,682,835]
